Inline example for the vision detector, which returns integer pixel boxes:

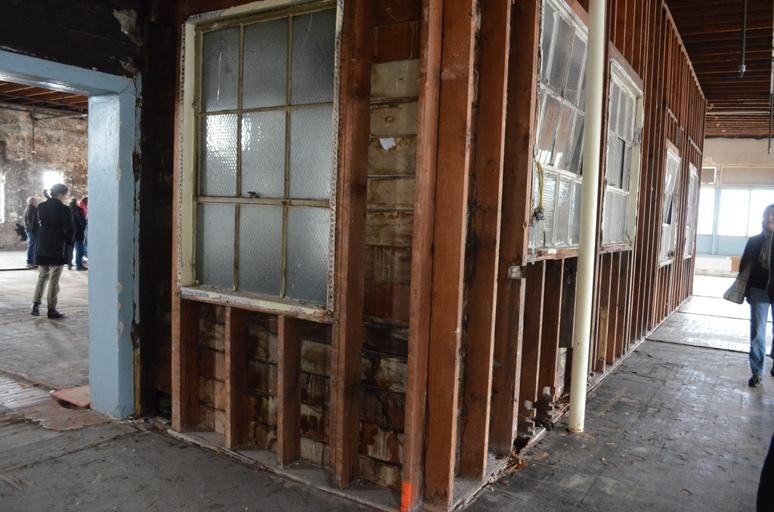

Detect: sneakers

[749,374,761,387]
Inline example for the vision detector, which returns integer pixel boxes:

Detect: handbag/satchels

[723,260,752,303]
[36,226,65,260]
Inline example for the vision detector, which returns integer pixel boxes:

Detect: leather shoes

[77,265,88,270]
[32,304,67,318]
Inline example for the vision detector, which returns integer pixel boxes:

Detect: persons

[23,183,87,319]
[722,204,774,388]
